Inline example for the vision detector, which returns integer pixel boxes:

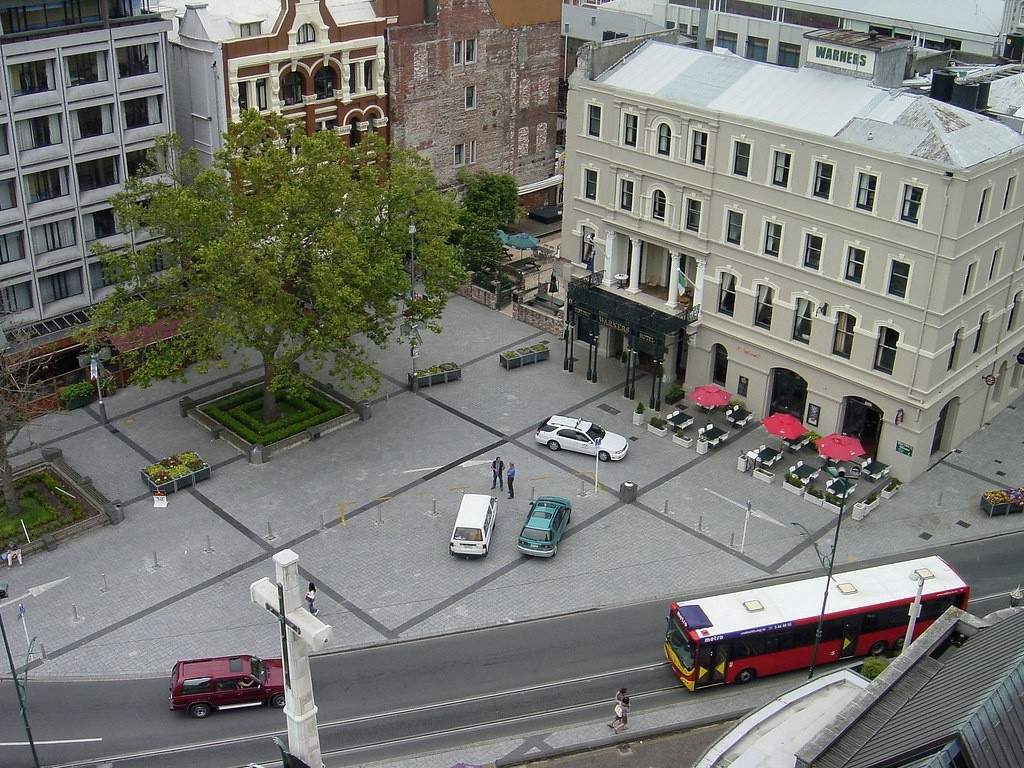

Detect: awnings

[4,331,108,367]
[108,314,212,353]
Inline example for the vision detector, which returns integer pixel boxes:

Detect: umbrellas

[497,229,511,244]
[814,433,866,461]
[761,412,809,451]
[688,385,734,421]
[549,269,559,298]
[507,233,540,258]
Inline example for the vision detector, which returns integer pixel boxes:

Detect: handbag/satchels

[305,595,312,602]
[614,704,622,718]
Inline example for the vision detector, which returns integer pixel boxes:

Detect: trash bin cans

[620,480,638,503]
[356,400,372,420]
[105,498,125,525]
[179,396,193,417]
[42,446,62,461]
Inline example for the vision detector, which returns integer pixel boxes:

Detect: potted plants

[696,437,708,455]
[100,374,117,397]
[406,361,461,388]
[728,400,755,421]
[664,382,685,406]
[647,416,668,437]
[58,380,95,411]
[671,428,694,449]
[862,490,879,513]
[632,401,646,426]
[499,342,551,371]
[881,477,902,499]
[821,491,848,515]
[782,472,805,497]
[803,484,825,507]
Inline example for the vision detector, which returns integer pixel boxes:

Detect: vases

[139,453,212,494]
[981,495,1024,517]
[752,467,776,484]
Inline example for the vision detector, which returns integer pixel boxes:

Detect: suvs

[169,655,289,719]
[449,493,498,559]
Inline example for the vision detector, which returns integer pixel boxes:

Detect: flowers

[982,487,1024,504]
[146,451,203,483]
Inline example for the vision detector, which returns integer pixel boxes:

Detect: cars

[535,415,629,462]
[517,495,572,557]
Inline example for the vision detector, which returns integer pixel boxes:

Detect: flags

[678,271,688,296]
[586,252,595,270]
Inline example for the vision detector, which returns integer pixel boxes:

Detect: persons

[235,679,254,689]
[491,456,506,492]
[606,687,631,734]
[507,462,515,499]
[306,583,319,617]
[6,542,22,567]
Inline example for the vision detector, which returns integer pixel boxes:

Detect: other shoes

[314,609,320,616]
[621,727,628,730]
[491,487,496,490]
[500,487,504,491]
[508,496,514,499]
[608,722,614,728]
[614,727,618,734]
[508,490,511,493]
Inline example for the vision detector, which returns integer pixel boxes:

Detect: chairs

[665,398,893,498]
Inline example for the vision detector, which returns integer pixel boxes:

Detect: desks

[615,273,629,290]
[728,407,751,428]
[783,434,807,446]
[791,463,817,479]
[757,446,780,462]
[828,478,854,495]
[864,460,888,475]
[668,412,693,432]
[701,427,726,441]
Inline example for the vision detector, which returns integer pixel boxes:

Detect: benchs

[0,542,45,565]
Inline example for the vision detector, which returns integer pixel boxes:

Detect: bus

[665,556,968,691]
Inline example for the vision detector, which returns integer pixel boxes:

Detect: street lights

[805,464,863,677]
[409,224,419,395]
[90,352,108,425]
[0,584,41,767]
[901,567,936,656]
